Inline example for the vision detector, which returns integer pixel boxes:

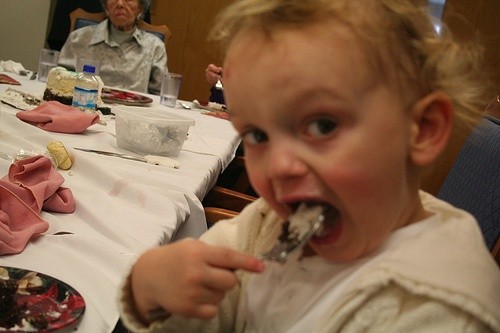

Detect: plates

[0,74,21,85]
[101,87,154,106]
[0,266,85,333]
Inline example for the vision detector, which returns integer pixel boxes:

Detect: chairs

[67,8,259,225]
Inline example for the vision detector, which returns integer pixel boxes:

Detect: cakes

[277,202,325,244]
[0,278,48,332]
[43,66,104,109]
[208,86,228,111]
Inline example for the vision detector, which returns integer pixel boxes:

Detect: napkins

[0,154,76,257]
[15,99,100,135]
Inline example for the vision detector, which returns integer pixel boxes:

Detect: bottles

[71,64,99,114]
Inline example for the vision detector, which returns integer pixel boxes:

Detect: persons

[118,0,500,333]
[57,0,172,96]
[430,0,500,251]
[205,63,225,105]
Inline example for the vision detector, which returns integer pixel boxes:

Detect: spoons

[145,212,328,320]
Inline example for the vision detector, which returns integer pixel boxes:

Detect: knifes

[73,147,180,169]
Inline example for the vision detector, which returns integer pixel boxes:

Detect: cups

[159,72,183,107]
[38,48,60,82]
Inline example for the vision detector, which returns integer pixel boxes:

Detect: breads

[48,140,72,170]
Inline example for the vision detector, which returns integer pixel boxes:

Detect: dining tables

[0,60,243,333]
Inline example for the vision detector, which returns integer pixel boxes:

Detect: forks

[215,78,223,90]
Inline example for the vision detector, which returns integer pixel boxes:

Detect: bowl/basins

[110,105,196,157]
